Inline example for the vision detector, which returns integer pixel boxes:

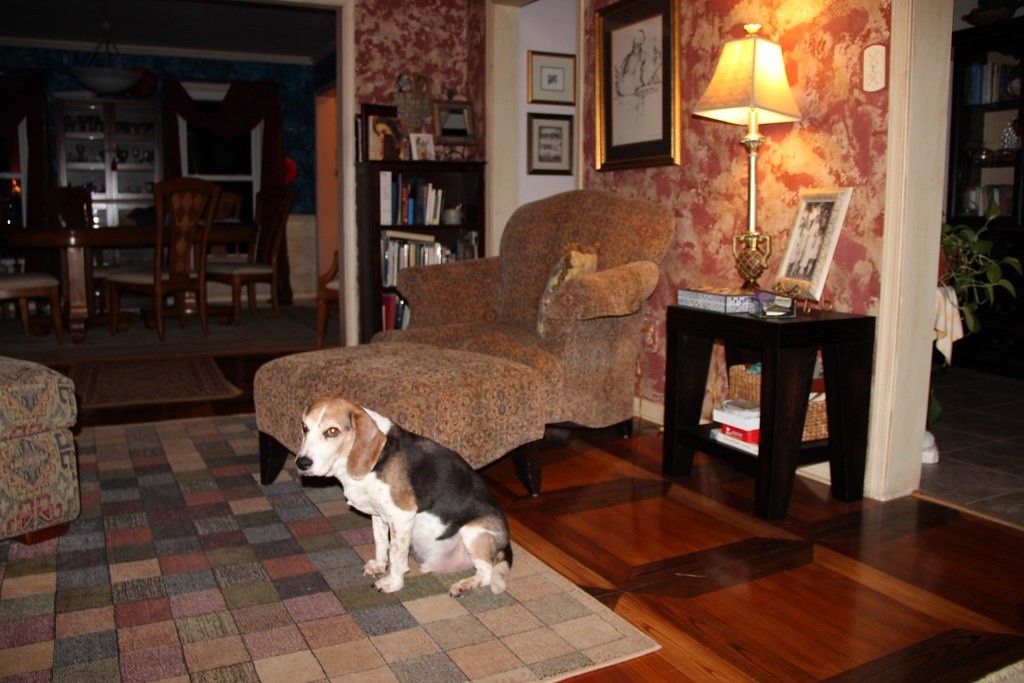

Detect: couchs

[395,188,679,441]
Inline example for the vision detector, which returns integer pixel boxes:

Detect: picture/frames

[592,0,680,172]
[771,186,854,301]
[527,112,574,177]
[361,102,400,163]
[430,98,478,144]
[527,49,577,107]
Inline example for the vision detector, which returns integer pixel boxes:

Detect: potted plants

[942,201,1022,340]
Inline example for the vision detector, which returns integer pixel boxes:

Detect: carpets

[0,311,323,409]
[0,412,661,683]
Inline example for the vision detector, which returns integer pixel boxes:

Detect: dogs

[294,396,514,596]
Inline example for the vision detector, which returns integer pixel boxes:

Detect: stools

[0,355,79,541]
[254,342,544,521]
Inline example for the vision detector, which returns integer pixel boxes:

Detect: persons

[373,117,403,158]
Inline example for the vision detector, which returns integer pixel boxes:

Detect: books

[382,294,410,331]
[966,63,1013,103]
[711,402,758,454]
[971,185,1013,216]
[380,171,443,225]
[380,230,478,286]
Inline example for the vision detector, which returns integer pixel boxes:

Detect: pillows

[537,240,598,338]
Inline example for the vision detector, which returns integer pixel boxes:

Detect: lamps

[691,24,802,293]
[79,17,145,94]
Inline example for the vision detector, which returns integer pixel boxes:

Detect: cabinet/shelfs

[955,16,1024,380]
[55,99,165,266]
[355,160,488,343]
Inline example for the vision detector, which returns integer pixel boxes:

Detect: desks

[1,221,256,342]
[656,304,876,517]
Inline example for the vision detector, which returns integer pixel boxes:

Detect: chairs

[0,176,298,346]
